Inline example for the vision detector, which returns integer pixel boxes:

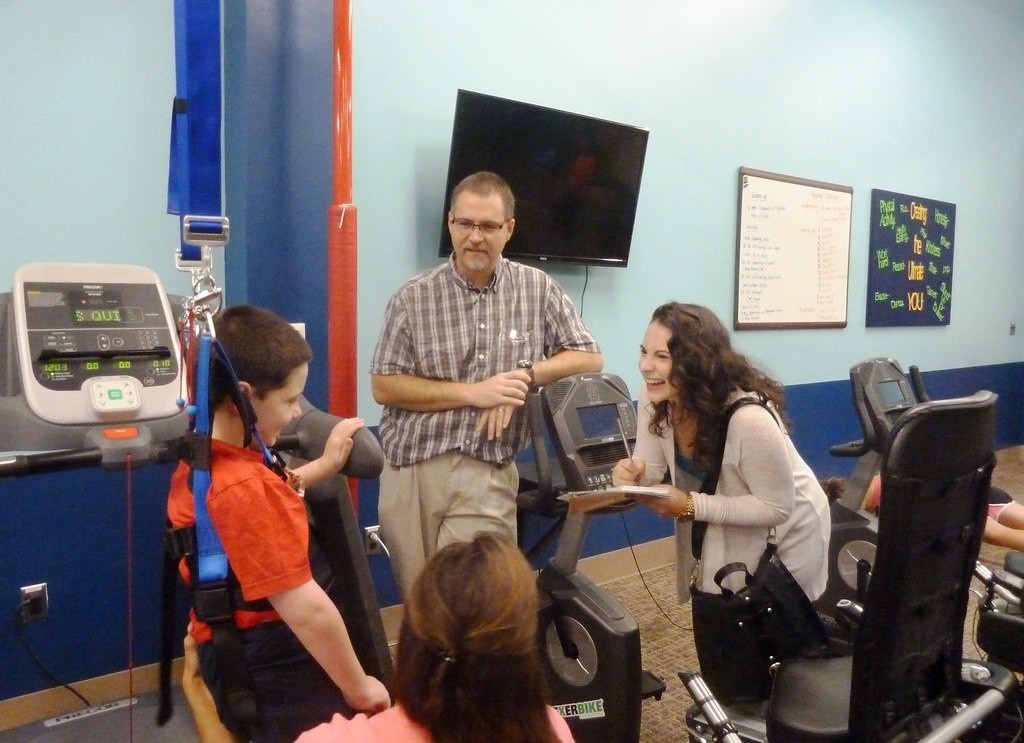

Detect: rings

[498,409,505,412]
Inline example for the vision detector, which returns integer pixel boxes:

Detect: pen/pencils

[615,414,640,484]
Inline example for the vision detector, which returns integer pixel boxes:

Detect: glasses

[451,208,506,235]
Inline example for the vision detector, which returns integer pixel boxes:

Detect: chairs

[769,388,999,743]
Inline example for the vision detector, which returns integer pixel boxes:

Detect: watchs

[676,496,694,523]
[517,360,535,387]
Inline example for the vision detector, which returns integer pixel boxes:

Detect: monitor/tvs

[437,88,651,269]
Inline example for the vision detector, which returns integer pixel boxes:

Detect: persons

[368,169,605,609]
[291,530,577,743]
[816,471,1024,552]
[165,303,391,743]
[612,303,833,707]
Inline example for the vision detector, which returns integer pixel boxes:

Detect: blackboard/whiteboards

[731,165,852,331]
[863,188,957,327]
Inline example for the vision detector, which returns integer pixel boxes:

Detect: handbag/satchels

[690,543,829,706]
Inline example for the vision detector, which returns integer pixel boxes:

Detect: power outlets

[20,583,50,627]
